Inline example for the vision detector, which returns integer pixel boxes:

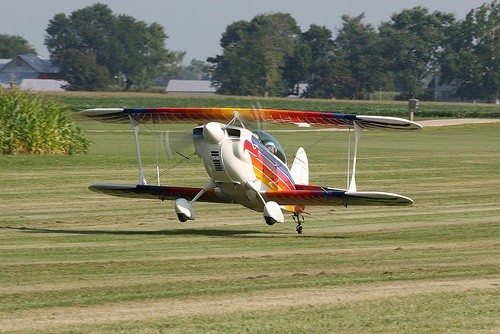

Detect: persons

[264,141,278,154]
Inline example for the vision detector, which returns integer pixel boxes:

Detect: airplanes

[81,107,423,234]
